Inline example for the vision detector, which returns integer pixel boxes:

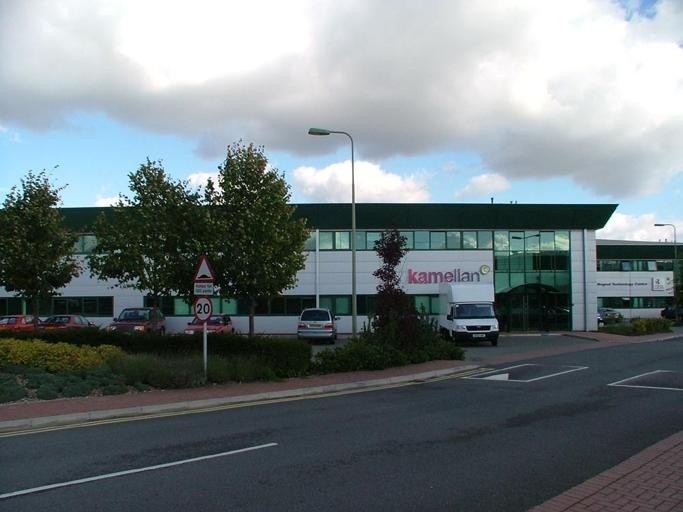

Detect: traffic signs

[194,296,213,321]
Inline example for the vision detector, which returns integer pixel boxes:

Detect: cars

[0,314,42,333]
[37,314,97,333]
[184,313,235,335]
[297,307,340,344]
[597,310,624,328]
[661,306,683,321]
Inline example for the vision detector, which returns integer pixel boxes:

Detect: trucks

[439,282,499,346]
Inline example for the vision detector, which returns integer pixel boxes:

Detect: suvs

[105,308,166,335]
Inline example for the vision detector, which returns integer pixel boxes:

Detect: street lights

[654,223,677,259]
[512,234,540,331]
[307,128,357,342]
